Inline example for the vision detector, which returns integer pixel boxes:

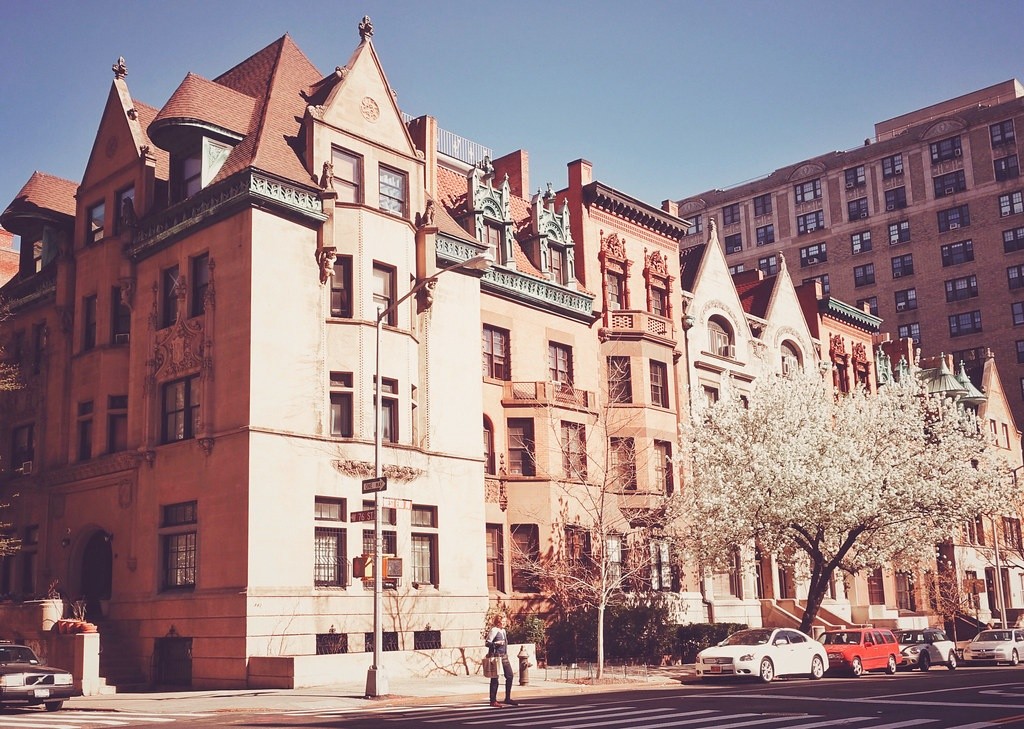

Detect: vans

[816,627,903,677]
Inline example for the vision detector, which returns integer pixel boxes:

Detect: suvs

[889,626,958,671]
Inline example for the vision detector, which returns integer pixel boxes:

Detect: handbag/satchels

[482,657,504,678]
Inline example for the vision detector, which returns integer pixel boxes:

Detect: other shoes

[490,701,502,707]
[504,699,518,705]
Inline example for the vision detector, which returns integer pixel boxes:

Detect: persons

[1014,613,1024,627]
[484,614,518,707]
[986,623,993,630]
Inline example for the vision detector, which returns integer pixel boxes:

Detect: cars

[0,640,73,712]
[963,628,1024,665]
[696,626,829,682]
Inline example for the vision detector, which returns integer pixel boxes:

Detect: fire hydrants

[518,645,533,686]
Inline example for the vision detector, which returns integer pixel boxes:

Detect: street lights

[348,253,495,699]
